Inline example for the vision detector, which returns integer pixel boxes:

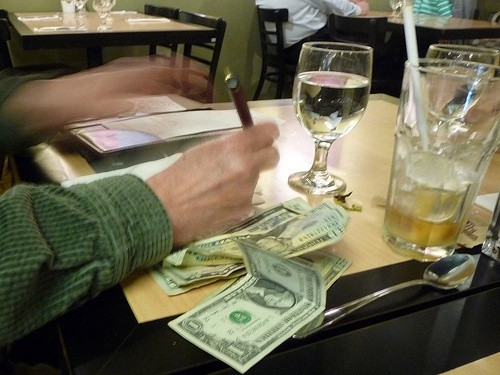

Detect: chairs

[0,4,389,104]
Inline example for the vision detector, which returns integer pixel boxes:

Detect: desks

[10,94,500,375]
[361,10,500,40]
[7,11,216,69]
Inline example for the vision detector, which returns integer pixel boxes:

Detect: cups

[382,58,500,262]
[425,44,499,66]
[482,192,500,262]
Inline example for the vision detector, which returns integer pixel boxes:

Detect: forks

[374,196,476,236]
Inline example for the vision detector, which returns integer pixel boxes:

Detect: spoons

[292,254,476,338]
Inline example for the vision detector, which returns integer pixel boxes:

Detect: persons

[382,0,452,90]
[256,1,370,69]
[0,57,280,375]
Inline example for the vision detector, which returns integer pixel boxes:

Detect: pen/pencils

[227,77,253,128]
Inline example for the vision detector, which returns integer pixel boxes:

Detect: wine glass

[69,0,88,31]
[288,41,373,195]
[388,0,401,17]
[91,0,117,30]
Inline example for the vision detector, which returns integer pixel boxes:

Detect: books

[59,93,279,156]
